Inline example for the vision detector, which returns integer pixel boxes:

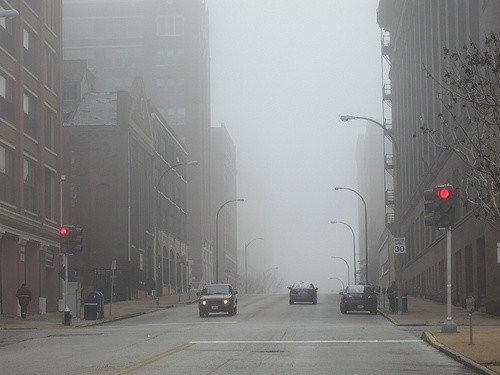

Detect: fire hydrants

[64,306,72,325]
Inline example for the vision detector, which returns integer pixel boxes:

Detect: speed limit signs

[394,238,406,254]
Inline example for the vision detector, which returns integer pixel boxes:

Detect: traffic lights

[423,184,453,227]
[60,226,83,253]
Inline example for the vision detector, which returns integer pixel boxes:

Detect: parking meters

[465,293,477,344]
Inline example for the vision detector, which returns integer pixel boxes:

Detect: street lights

[332,257,349,284]
[331,221,356,284]
[215,198,244,282]
[330,278,344,294]
[341,115,403,314]
[245,238,265,293]
[335,186,368,284]
[156,160,200,305]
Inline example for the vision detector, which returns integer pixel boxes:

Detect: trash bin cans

[83,303,98,320]
[395,297,407,312]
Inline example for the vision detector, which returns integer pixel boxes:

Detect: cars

[339,285,378,315]
[287,282,318,304]
[198,284,239,317]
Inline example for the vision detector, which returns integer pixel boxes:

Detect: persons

[17,283,33,318]
[387,281,398,312]
